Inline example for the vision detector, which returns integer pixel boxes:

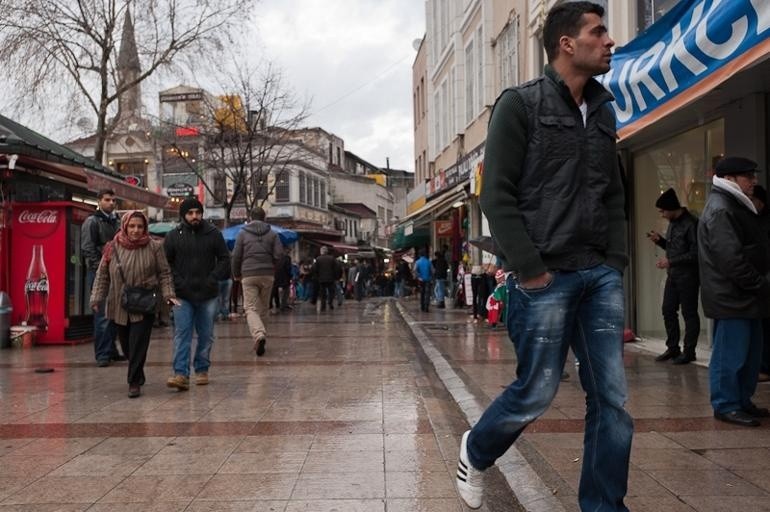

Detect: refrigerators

[2,201,98,345]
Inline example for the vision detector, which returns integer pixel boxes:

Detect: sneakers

[268,305,295,314]
[97,355,126,367]
[196,373,209,384]
[255,337,265,355]
[457,429,486,508]
[309,299,343,311]
[167,375,189,390]
[215,310,246,319]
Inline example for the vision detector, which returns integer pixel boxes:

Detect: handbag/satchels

[122,285,160,314]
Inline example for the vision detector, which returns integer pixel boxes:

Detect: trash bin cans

[0,291,13,348]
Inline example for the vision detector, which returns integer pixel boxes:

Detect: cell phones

[646,231,657,241]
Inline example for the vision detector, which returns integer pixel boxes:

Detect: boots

[434,301,445,308]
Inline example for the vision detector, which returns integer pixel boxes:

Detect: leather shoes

[128,370,145,396]
[713,401,770,426]
[656,348,697,364]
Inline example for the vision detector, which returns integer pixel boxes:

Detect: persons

[231,206,284,356]
[78,187,124,368]
[162,196,230,391]
[454,1,637,511]
[89,210,182,400]
[269,242,450,313]
[470,265,489,324]
[646,187,701,365]
[694,155,769,426]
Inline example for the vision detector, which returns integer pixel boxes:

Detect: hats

[655,188,683,210]
[180,199,204,217]
[715,156,758,177]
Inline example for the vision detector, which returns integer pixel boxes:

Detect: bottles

[24,239,49,333]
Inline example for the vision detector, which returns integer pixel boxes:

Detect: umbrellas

[220,218,298,250]
[468,232,508,269]
